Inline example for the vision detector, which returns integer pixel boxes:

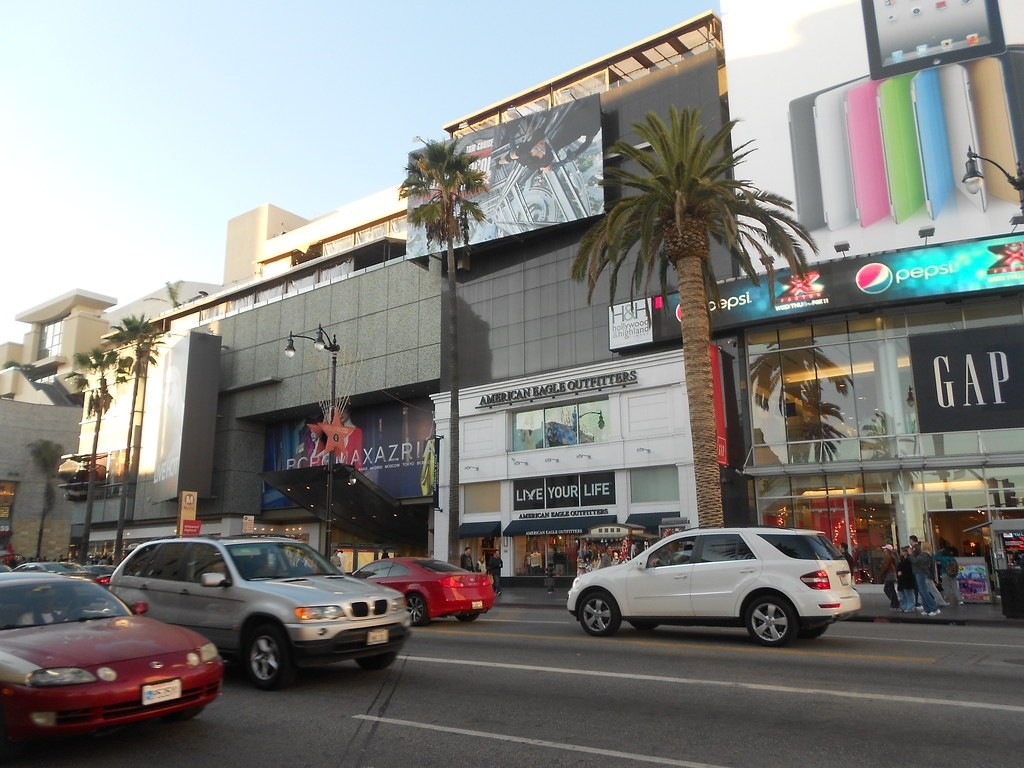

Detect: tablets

[787,0,1024,231]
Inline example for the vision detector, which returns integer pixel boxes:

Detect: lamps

[513,460,528,465]
[466,466,480,470]
[545,458,558,463]
[577,454,591,459]
[759,254,776,265]
[918,225,935,243]
[638,448,650,453]
[728,339,735,345]
[434,507,443,512]
[1009,213,1024,233]
[834,240,850,257]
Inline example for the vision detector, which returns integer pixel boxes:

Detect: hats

[882,544,894,551]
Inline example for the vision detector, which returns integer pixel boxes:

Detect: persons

[838,543,854,587]
[461,546,504,599]
[881,536,964,616]
[598,551,611,570]
[1,553,114,570]
[544,563,555,595]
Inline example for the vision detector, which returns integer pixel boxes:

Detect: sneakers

[921,608,941,617]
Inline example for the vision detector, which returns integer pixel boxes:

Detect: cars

[0,570,224,744]
[350,557,494,627]
[0,562,117,598]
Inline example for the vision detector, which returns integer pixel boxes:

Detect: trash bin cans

[994,567,1024,620]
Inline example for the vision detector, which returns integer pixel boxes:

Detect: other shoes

[497,592,502,597]
[898,606,915,612]
[914,603,924,609]
[937,603,951,607]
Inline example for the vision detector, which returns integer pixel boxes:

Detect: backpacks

[945,560,958,577]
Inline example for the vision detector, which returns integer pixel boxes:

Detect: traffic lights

[1003,478,1017,508]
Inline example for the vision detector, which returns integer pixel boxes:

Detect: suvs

[108,533,412,692]
[566,526,861,647]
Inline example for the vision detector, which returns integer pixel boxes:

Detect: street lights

[285,323,340,564]
[962,146,1024,216]
[573,411,605,443]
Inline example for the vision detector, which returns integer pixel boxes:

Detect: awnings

[459,521,501,538]
[503,515,617,536]
[625,512,679,529]
